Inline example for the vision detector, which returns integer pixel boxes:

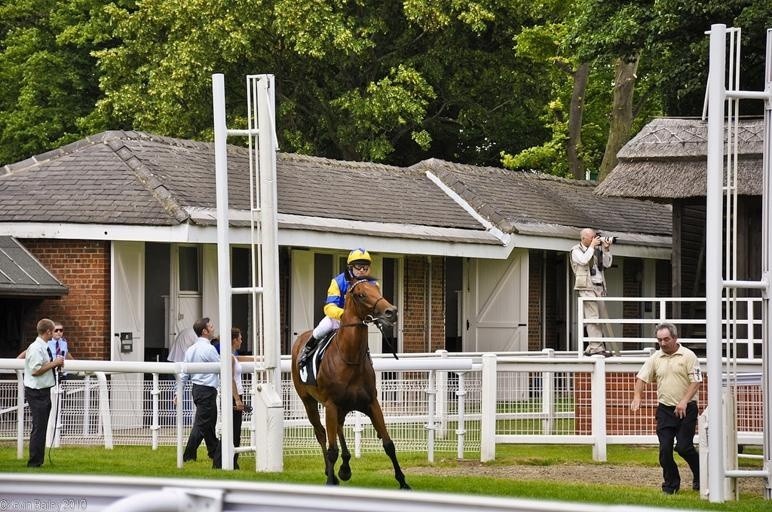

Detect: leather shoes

[583,352,592,357]
[593,352,614,358]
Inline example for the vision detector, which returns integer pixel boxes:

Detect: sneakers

[693,482,700,491]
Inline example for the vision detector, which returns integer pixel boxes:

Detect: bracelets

[50,360,56,369]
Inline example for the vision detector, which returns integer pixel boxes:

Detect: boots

[297,335,319,369]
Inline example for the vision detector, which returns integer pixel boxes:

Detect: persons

[630,321,704,494]
[15,321,75,383]
[294,247,373,368]
[166,328,198,428]
[570,228,615,358]
[22,317,64,468]
[211,328,245,470]
[171,317,222,462]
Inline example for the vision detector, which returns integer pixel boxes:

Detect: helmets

[347,248,372,281]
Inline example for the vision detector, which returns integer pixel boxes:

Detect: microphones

[55,350,66,372]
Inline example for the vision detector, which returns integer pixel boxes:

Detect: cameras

[593,231,619,250]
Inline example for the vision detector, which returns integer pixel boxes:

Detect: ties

[46,346,56,385]
[55,341,61,355]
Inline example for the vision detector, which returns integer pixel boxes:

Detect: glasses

[353,263,369,270]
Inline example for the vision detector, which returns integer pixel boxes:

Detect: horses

[291,268,413,490]
